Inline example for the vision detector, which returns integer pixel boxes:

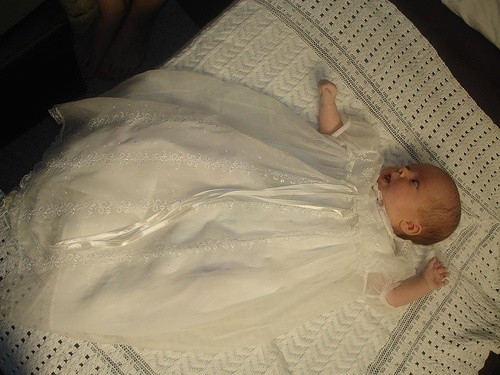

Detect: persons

[59,79,463,307]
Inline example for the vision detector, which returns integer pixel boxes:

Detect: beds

[1,0,500,375]
